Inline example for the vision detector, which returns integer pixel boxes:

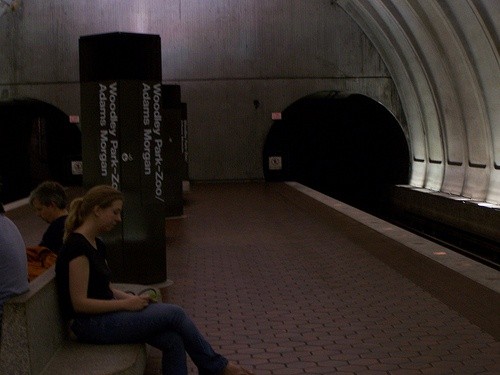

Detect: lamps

[272,112,281,120]
[70,115,80,124]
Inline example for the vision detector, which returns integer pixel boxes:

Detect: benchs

[23,254,164,375]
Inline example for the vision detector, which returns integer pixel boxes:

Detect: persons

[22,182,72,275]
[0,204,30,305]
[53,185,258,375]
